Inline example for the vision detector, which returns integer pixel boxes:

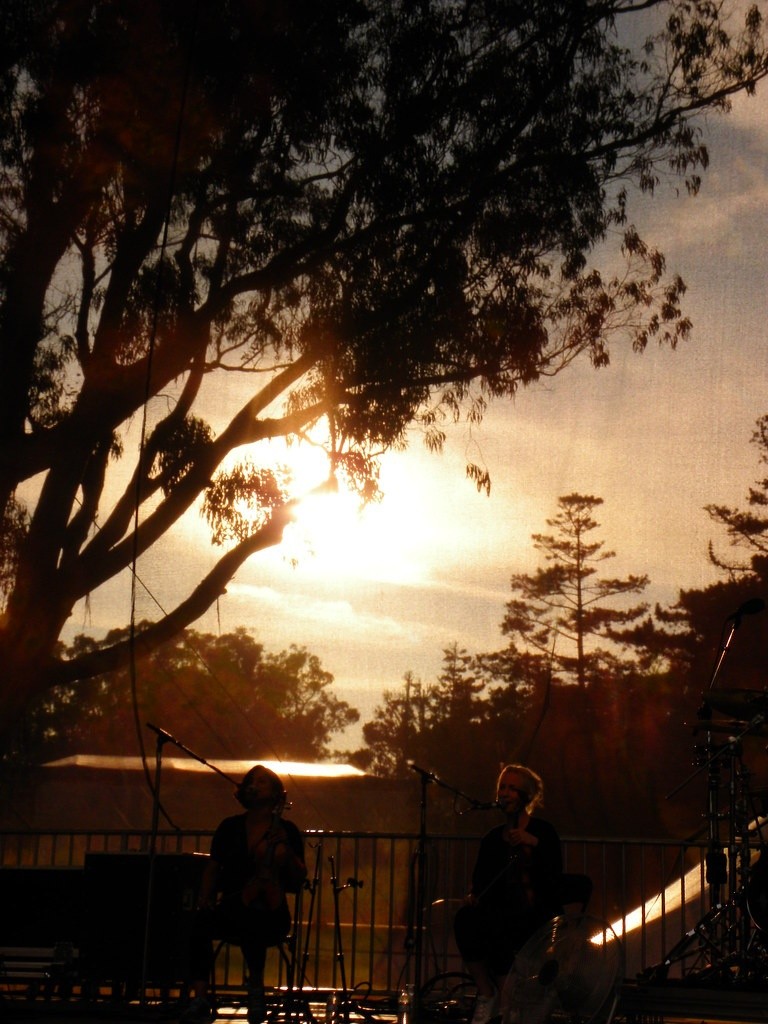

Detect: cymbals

[694,686,766,735]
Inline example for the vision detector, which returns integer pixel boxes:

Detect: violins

[240,788,292,915]
[497,809,535,919]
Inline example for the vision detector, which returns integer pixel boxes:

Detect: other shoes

[247,987,266,1023]
[179,1001,210,1023]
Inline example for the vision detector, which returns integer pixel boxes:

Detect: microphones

[727,598,765,621]
[246,785,258,796]
[470,801,509,811]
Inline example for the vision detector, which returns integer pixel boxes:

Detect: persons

[452,764,562,1024]
[192,765,307,1024]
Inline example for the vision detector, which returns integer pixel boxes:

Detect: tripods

[636,612,751,985]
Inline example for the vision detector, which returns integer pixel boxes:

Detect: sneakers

[471,982,498,1024]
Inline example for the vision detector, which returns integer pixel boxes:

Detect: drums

[746,857,768,932]
